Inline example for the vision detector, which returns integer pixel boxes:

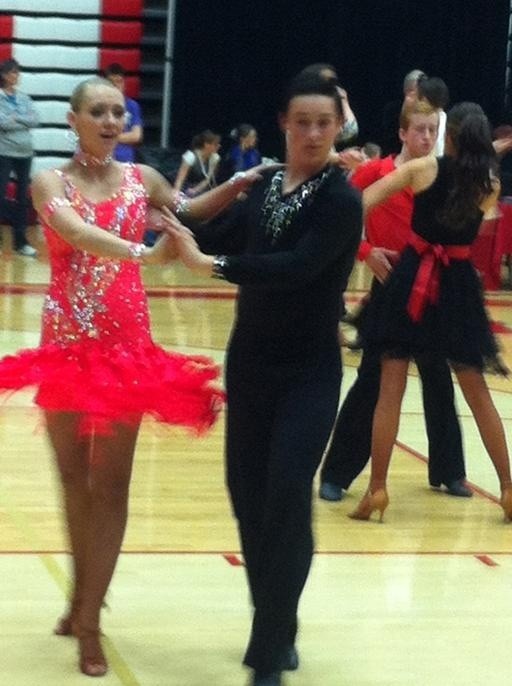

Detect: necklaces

[72,140,112,168]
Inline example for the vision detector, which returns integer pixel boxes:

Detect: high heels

[499,489,512,524]
[345,487,389,524]
[319,481,343,501]
[429,478,473,497]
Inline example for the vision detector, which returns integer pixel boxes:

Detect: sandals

[54,610,100,636]
[73,623,108,677]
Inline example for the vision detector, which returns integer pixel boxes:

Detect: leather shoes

[253,669,281,686]
[278,645,298,670]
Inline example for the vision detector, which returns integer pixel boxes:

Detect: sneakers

[12,244,39,256]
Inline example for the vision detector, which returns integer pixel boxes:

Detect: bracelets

[127,242,145,266]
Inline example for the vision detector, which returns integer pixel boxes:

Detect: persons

[163,72,363,686]
[0,57,38,257]
[101,63,159,246]
[0,79,288,678]
